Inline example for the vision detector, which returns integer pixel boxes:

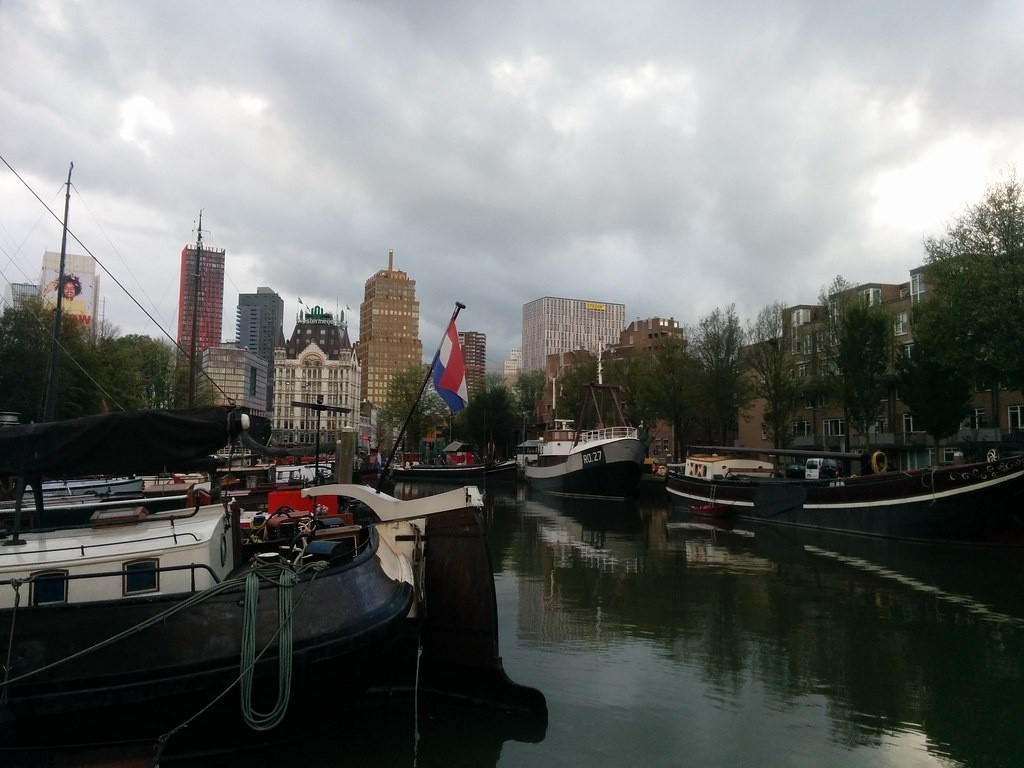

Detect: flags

[348,306,352,311]
[298,297,303,304]
[375,450,382,472]
[431,316,469,414]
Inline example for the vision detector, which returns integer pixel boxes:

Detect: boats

[515,439,540,479]
[664,446,1024,548]
[523,381,648,491]
[0,395,507,768]
[382,440,486,478]
[0,459,336,528]
[640,457,667,482]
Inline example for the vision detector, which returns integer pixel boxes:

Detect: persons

[42,274,89,329]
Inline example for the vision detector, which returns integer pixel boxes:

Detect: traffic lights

[363,436,368,440]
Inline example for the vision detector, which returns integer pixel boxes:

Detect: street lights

[314,395,324,486]
